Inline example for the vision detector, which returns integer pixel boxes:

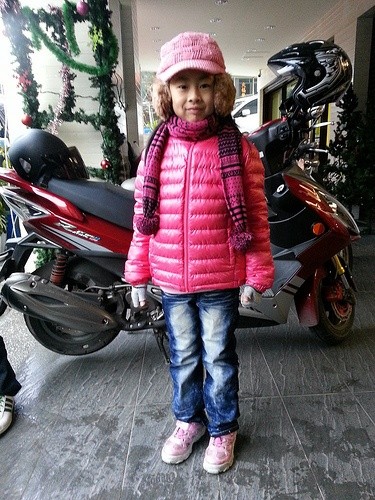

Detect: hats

[156,31,226,82]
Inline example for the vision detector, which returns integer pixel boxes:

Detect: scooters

[0,40,361,366]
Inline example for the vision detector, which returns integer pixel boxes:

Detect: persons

[0,335,22,435]
[125,32,274,475]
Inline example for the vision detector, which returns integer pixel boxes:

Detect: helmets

[8,130,90,183]
[268,38,353,108]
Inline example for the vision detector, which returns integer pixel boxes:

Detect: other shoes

[203,430,238,475]
[161,414,206,465]
[0,395,16,435]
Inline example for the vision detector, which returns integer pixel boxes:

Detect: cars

[231,93,260,136]
